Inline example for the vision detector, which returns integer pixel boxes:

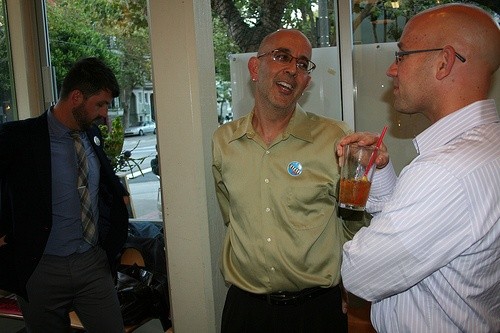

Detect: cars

[125,120,157,137]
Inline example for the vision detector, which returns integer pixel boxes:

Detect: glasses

[395,48,465,63]
[257,49,317,74]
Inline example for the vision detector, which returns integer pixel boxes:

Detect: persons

[0,57,130,333]
[211,29,373,333]
[340,2,500,333]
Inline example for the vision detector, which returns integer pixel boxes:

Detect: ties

[68,129,96,247]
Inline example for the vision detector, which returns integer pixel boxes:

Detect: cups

[338,142,380,211]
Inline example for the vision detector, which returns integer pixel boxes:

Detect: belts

[251,287,327,307]
